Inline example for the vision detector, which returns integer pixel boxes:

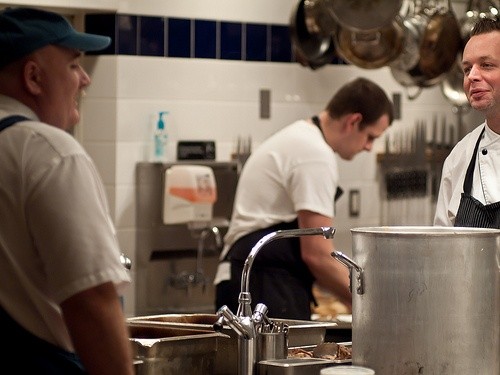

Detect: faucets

[216,225,336,341]
[181,224,221,297]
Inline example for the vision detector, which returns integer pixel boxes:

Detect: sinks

[126,313,338,372]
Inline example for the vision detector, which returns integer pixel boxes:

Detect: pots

[331,226,500,375]
[291,0,500,107]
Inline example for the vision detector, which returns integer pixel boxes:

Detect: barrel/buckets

[256,333,287,361]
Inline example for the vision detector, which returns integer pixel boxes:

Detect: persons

[433,18,500,232]
[0,7,133,375]
[214,77,395,320]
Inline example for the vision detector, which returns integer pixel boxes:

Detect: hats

[1,7,112,65]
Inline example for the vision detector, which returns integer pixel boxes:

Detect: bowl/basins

[256,342,352,375]
[320,366,375,375]
